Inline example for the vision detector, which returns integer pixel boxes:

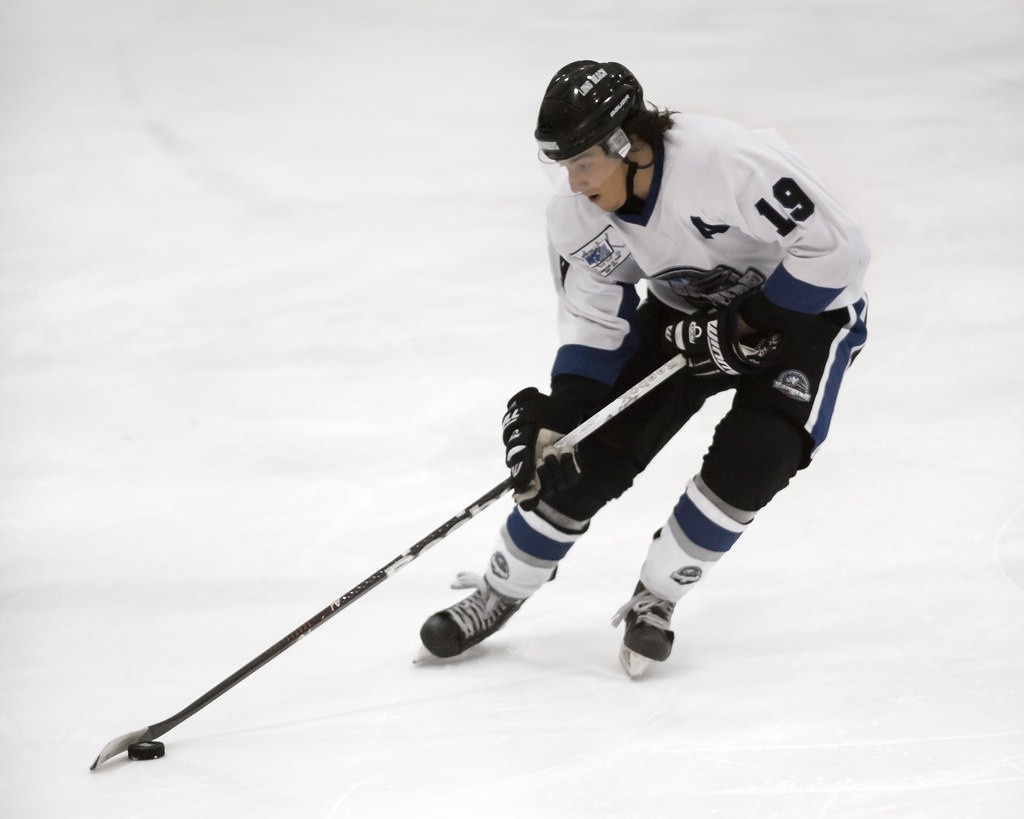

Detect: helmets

[534,59,642,160]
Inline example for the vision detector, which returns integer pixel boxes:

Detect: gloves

[665,306,783,381]
[500,384,595,510]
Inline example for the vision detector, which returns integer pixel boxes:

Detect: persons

[418,57,874,664]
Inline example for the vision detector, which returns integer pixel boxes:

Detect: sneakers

[616,580,676,680]
[412,574,526,667]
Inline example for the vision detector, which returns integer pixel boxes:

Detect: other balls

[128,742,166,761]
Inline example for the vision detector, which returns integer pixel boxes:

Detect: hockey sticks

[90,353,687,772]
